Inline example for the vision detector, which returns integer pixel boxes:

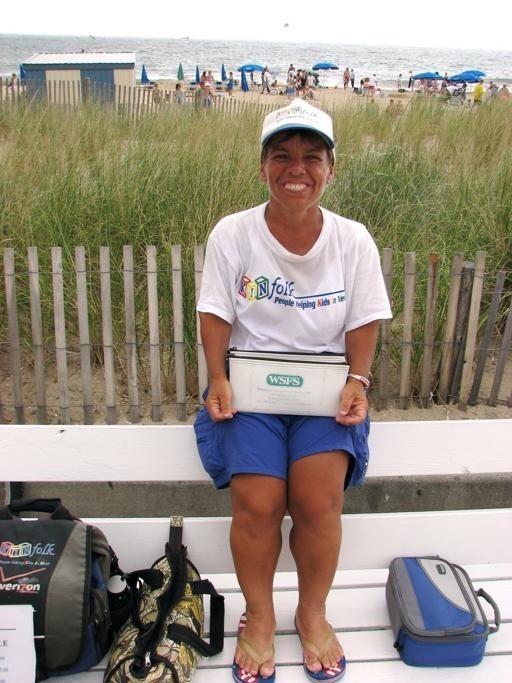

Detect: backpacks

[0,497,125,683]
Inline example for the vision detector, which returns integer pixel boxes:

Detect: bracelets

[347,374,370,390]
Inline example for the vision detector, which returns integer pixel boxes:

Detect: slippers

[232,612,275,683]
[294,615,346,683]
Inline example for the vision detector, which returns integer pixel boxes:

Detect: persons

[343,68,378,97]
[260,64,316,99]
[398,71,510,104]
[194,99,394,683]
[153,70,234,105]
[8,73,18,97]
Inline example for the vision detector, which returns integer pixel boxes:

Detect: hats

[260,99,335,150]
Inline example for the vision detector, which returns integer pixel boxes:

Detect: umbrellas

[196,65,199,83]
[222,63,227,81]
[312,63,339,70]
[141,64,149,83]
[178,62,183,80]
[242,65,249,92]
[237,64,266,72]
[20,64,26,85]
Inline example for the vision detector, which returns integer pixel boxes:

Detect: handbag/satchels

[227,349,351,418]
[385,555,500,667]
[103,555,225,683]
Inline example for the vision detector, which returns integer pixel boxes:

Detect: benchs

[1,417,511,677]
